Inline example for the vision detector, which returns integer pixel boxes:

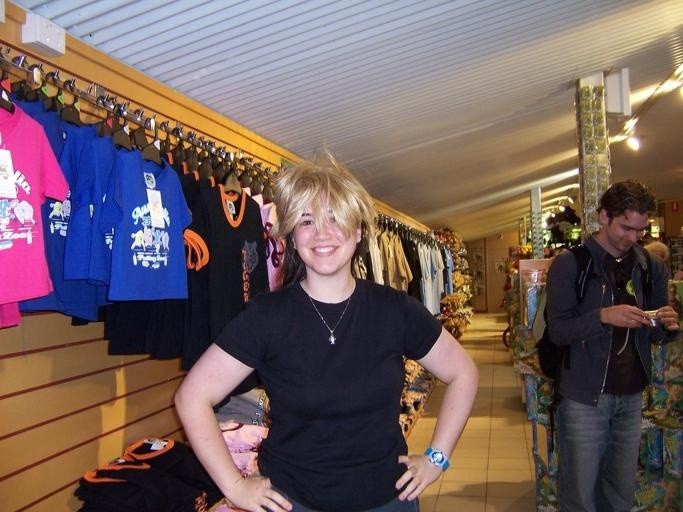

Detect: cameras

[641,310,660,330]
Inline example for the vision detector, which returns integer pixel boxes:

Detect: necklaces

[305,290,352,345]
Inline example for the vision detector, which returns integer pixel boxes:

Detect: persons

[173,160,481,512]
[643,241,670,263]
[545,180,680,512]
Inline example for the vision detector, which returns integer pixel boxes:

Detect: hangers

[0,50,438,249]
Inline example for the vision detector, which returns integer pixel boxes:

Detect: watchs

[423,447,449,472]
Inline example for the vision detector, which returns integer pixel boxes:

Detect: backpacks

[535,247,651,379]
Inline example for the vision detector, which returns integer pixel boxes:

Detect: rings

[631,319,636,325]
